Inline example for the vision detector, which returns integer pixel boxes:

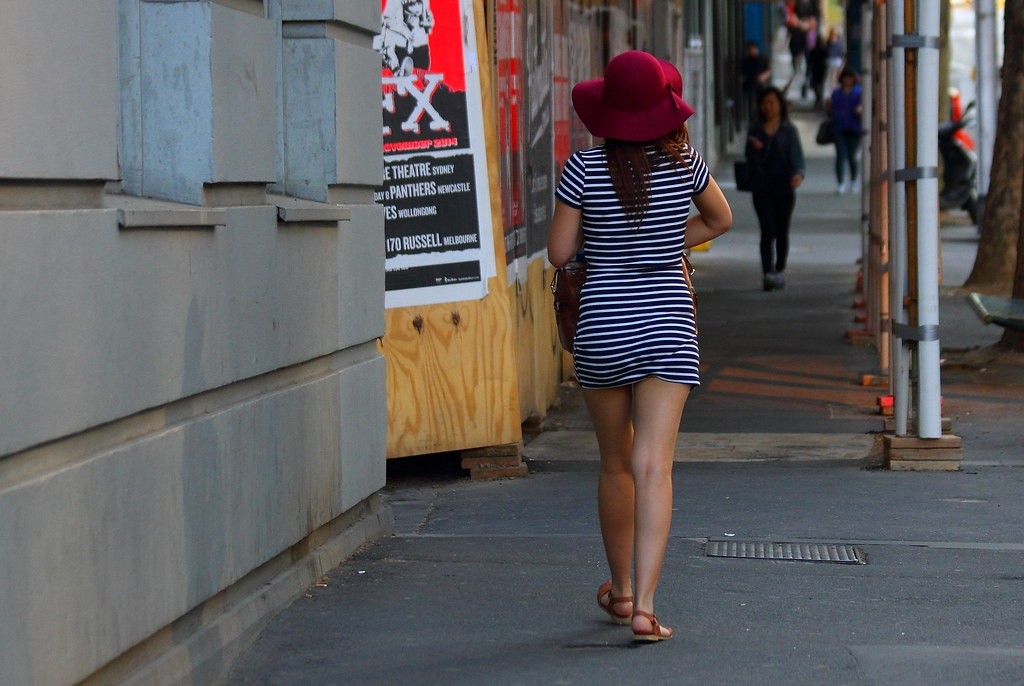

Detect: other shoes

[764,273,776,290]
[775,271,786,289]
[836,183,845,194]
[850,181,859,194]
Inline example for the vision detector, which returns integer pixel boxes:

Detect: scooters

[937,101,987,226]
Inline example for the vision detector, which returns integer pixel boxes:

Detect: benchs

[965,292,1024,330]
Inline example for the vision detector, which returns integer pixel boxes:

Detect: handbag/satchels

[550,254,700,356]
[816,106,836,145]
[733,160,762,191]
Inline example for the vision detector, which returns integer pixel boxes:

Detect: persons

[825,67,864,196]
[547,48,734,641]
[741,0,848,129]
[743,85,805,291]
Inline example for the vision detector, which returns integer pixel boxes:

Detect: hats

[571,49,696,143]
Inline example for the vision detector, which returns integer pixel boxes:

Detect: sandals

[597,581,634,626]
[629,611,674,641]
